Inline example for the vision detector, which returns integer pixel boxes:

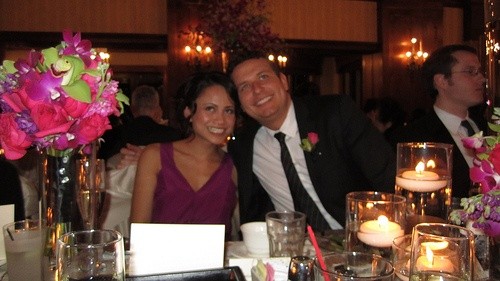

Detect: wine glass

[76,159,106,230]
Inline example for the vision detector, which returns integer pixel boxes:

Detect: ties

[274,132,332,233]
[461,120,476,138]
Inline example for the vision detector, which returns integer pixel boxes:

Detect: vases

[38,143,103,279]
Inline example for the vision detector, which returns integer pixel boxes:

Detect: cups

[56,229,126,281]
[313,251,395,281]
[395,143,452,234]
[2,219,40,281]
[240,222,269,258]
[392,234,412,281]
[409,223,475,281]
[345,192,406,262]
[265,210,306,257]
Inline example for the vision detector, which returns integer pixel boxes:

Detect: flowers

[0,29,130,161]
[448,106,500,238]
[298,132,320,152]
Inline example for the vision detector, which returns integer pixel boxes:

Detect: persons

[117,49,397,246]
[384,44,497,198]
[0,84,277,231]
[129,72,242,242]
[362,96,406,142]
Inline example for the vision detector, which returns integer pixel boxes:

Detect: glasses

[441,68,483,78]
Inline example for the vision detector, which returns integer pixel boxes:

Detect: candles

[344,141,474,281]
